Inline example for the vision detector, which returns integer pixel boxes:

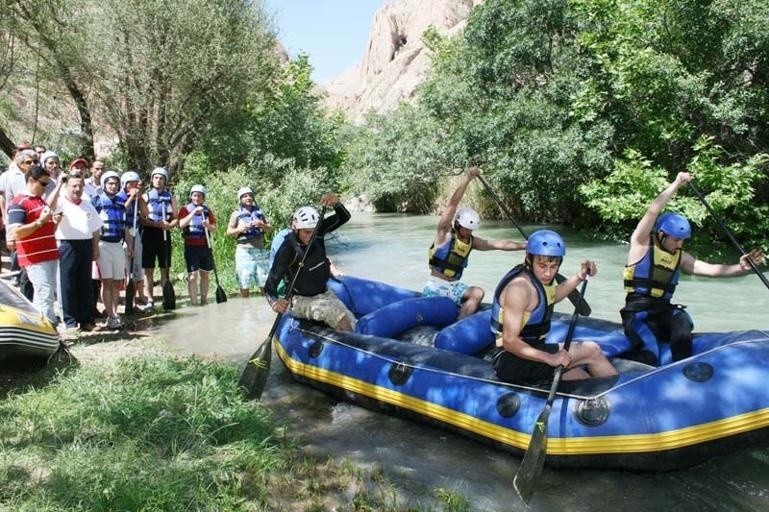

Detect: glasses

[25,160,38,165]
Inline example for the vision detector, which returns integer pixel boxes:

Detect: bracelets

[577,272,583,280]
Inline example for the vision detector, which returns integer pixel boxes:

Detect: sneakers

[67,302,153,332]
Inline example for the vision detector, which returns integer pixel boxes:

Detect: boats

[271,275,769,468]
[0,279,60,364]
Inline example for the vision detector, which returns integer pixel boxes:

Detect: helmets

[527,230,565,256]
[41,152,254,204]
[656,213,691,238]
[292,207,320,230]
[456,208,480,230]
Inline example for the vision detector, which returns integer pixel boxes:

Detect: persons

[619,171,765,368]
[8,165,62,322]
[178,184,214,302]
[489,228,619,384]
[263,192,359,334]
[226,187,271,299]
[117,171,149,316]
[46,174,103,333]
[421,167,528,320]
[91,170,127,328]
[0,143,148,304]
[141,168,178,309]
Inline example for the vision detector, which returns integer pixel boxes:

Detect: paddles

[200,210,227,303]
[513,268,590,508]
[125,193,139,314]
[238,201,328,402]
[158,196,176,309]
[476,174,593,316]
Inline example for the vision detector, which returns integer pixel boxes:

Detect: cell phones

[55,211,63,215]
